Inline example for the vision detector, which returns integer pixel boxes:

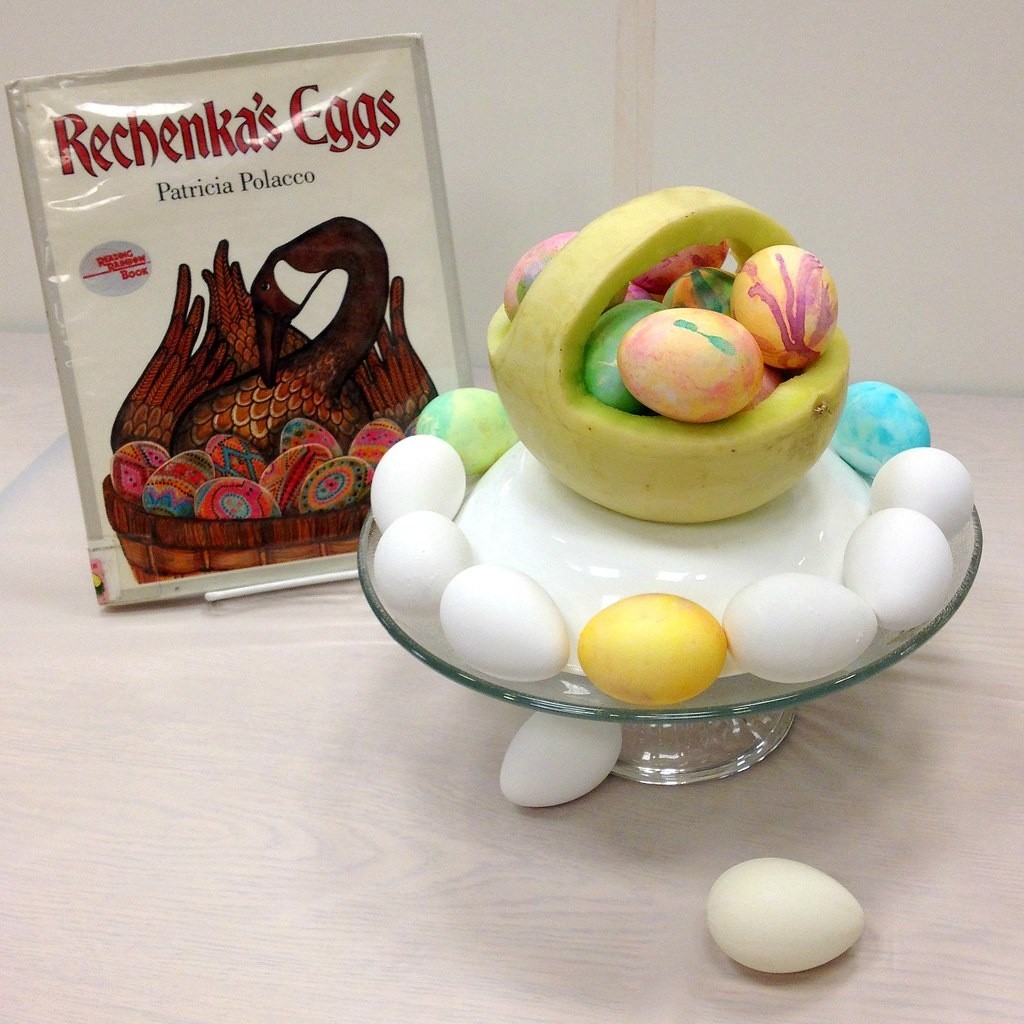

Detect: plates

[354,472,983,787]
